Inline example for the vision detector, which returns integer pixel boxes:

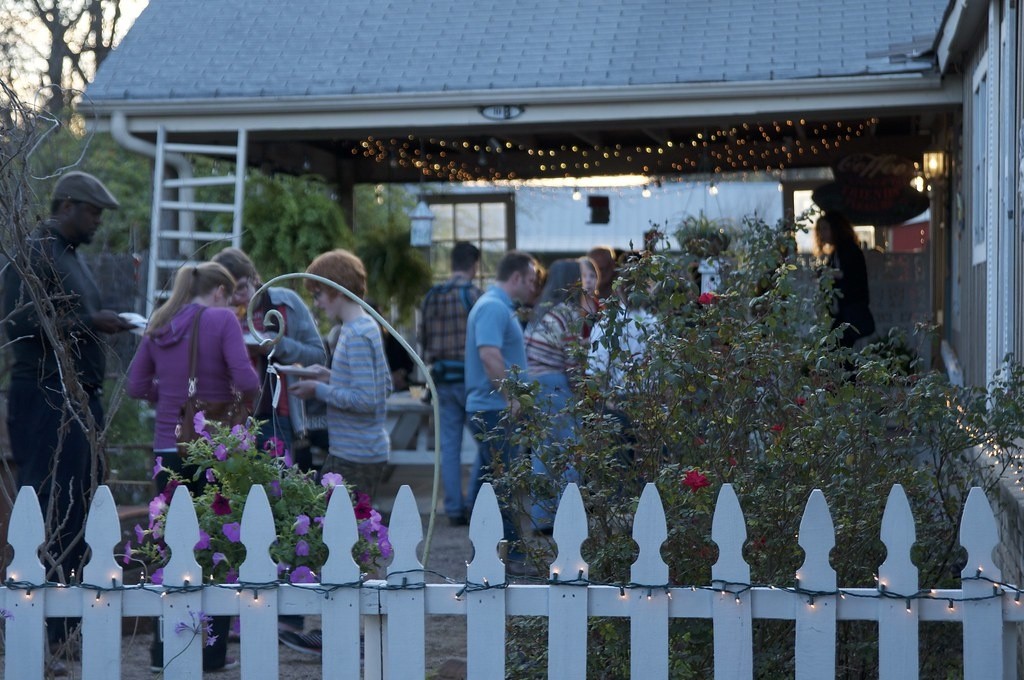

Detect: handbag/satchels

[175,398,252,456]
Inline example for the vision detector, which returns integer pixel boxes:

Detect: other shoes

[532,526,553,537]
[214,658,240,671]
[51,639,83,663]
[279,627,321,655]
[44,652,67,678]
[449,508,472,525]
[359,635,365,663]
[504,558,538,578]
[149,664,165,671]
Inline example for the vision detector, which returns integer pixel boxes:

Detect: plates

[275,365,324,376]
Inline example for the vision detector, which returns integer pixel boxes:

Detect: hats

[51,170,120,209]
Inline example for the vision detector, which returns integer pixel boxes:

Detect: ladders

[140,122,247,422]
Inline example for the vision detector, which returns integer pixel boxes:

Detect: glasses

[232,275,252,297]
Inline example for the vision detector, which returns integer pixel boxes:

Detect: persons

[212,247,326,642]
[463,250,537,577]
[125,260,261,672]
[304,300,414,452]
[816,213,877,384]
[685,228,738,303]
[416,241,485,526]
[4,172,138,676]
[522,248,700,536]
[279,249,390,665]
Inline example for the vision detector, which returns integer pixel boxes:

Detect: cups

[409,386,422,400]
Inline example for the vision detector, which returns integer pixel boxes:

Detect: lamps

[921,133,949,189]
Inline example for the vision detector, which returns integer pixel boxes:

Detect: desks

[377,391,479,484]
